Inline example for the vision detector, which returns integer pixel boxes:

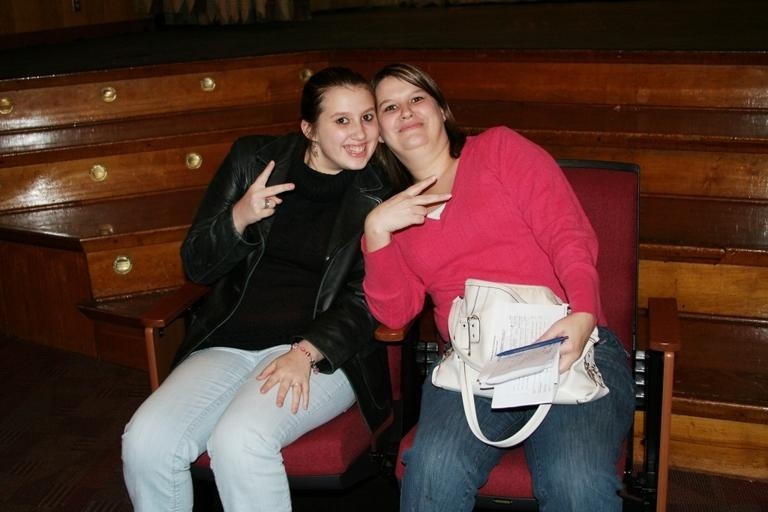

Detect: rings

[264,199,269,209]
[292,382,302,389]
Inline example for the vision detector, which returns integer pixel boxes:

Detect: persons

[116,64,427,511]
[357,57,642,511]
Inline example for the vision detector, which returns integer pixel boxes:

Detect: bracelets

[291,342,318,370]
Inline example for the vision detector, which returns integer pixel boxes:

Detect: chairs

[139,283,410,510]
[373,159,682,511]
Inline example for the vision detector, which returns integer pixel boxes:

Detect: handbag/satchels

[431,278,609,406]
